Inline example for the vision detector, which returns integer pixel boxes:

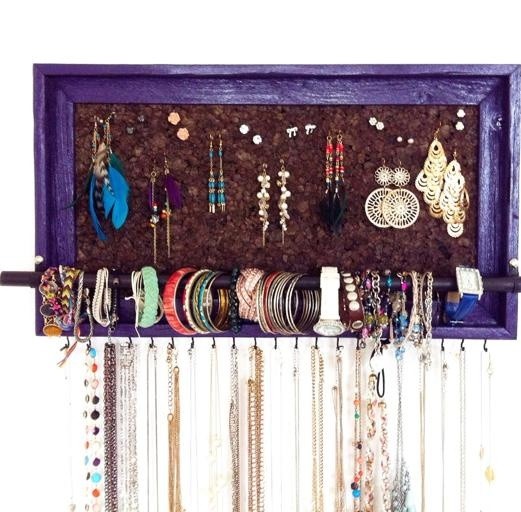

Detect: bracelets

[131,266,228,336]
[39,264,120,337]
[341,269,433,345]
[228,269,321,336]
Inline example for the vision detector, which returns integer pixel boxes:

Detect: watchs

[444,263,484,324]
[313,265,346,336]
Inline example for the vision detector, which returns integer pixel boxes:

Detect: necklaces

[295,346,409,512]
[85,344,181,512]
[421,348,492,512]
[189,345,265,512]
